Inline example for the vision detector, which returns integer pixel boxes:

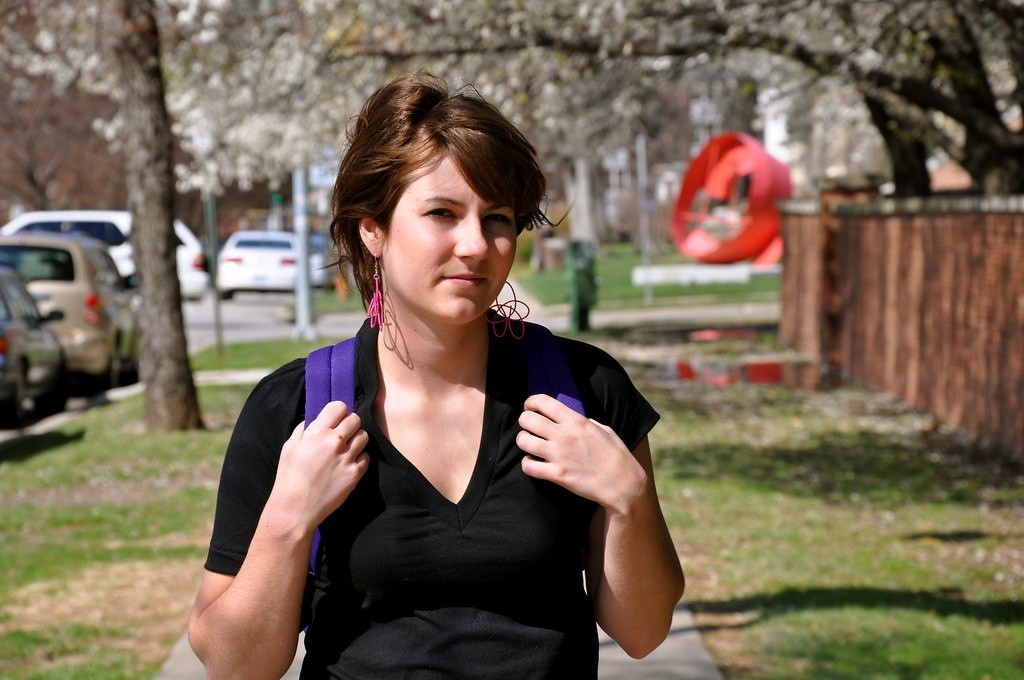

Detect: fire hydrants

[336,266,350,297]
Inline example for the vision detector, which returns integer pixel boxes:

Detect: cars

[0,264,69,426]
[215,231,300,299]
[309,236,334,289]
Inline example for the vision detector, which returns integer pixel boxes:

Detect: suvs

[0,232,141,388]
[0,208,212,304]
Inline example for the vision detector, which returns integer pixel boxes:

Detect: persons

[187,68,685,680]
